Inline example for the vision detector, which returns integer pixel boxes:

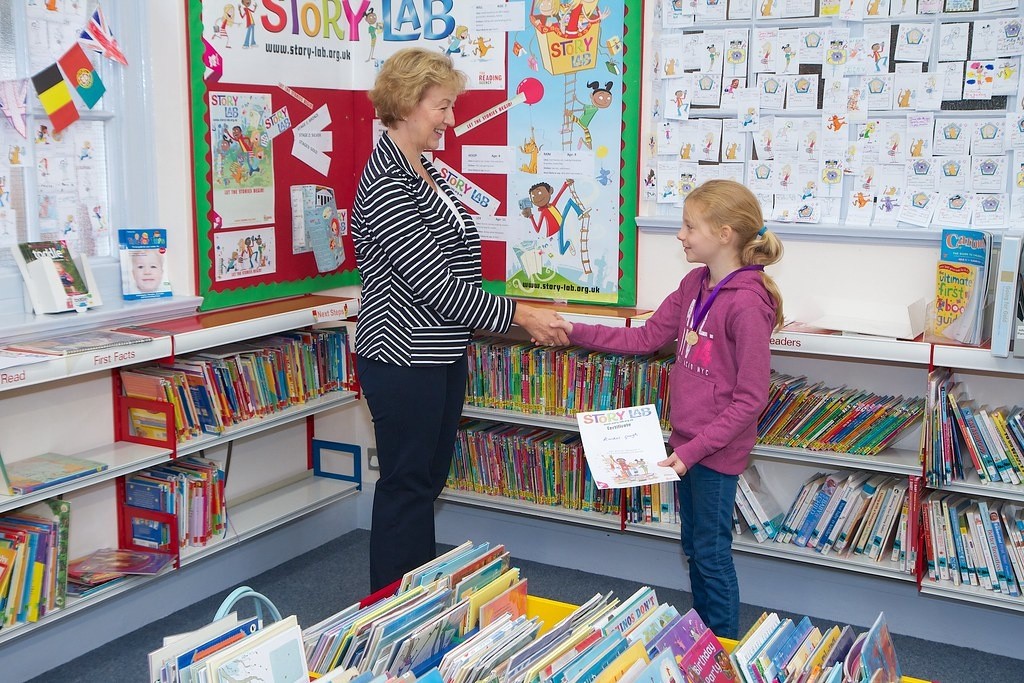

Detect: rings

[549,341,554,346]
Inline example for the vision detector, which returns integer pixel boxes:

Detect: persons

[352,49,569,593]
[532,180,785,640]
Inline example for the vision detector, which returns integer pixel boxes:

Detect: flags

[31,63,80,132]
[58,42,106,109]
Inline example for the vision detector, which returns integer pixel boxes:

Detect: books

[119,230,173,300]
[1,228,1024,683]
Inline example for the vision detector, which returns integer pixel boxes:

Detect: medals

[687,331,698,345]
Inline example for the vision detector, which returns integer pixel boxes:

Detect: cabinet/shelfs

[0,294,360,683]
[360,284,1024,612]
